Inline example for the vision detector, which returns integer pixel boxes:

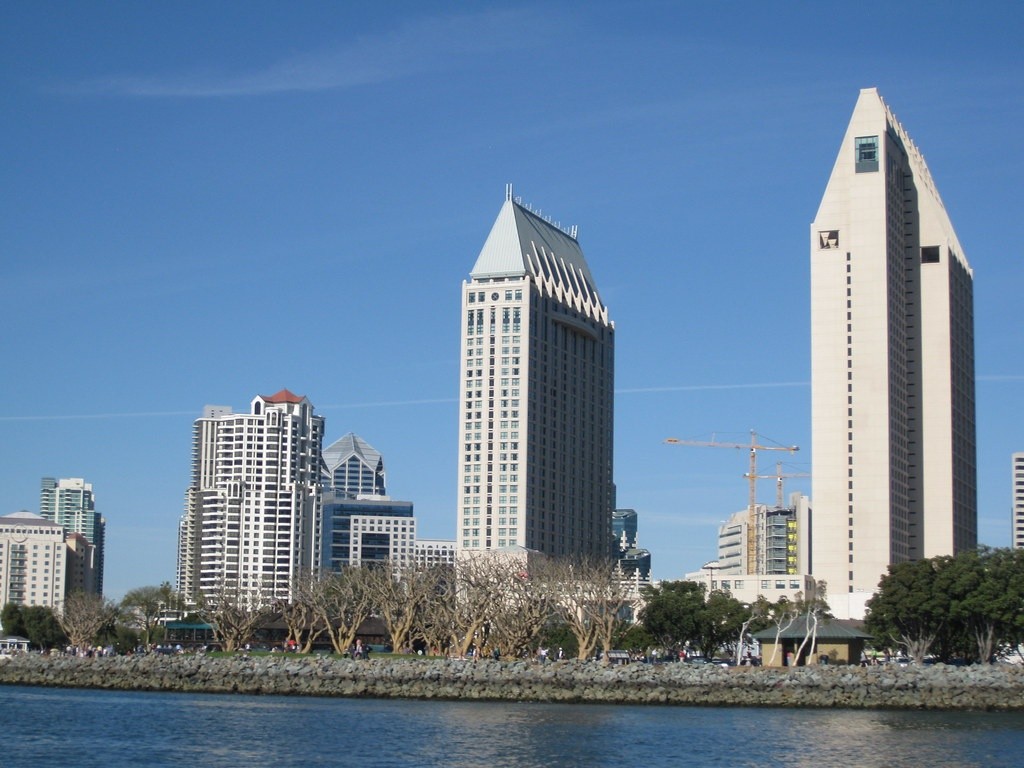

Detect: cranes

[664,429,812,576]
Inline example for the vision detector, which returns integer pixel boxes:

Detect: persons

[64,635,911,666]
[559,647,564,661]
